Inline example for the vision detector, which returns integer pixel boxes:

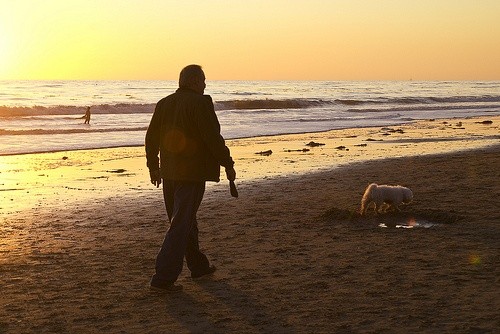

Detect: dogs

[360,184,413,214]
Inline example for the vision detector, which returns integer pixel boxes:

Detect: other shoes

[150,279,183,293]
[191,265,217,277]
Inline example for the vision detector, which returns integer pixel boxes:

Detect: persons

[146,65,236,292]
[82,107,91,124]
